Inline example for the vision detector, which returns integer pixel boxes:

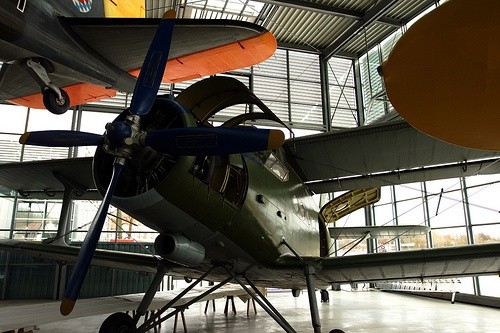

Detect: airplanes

[1,1,499,333]
